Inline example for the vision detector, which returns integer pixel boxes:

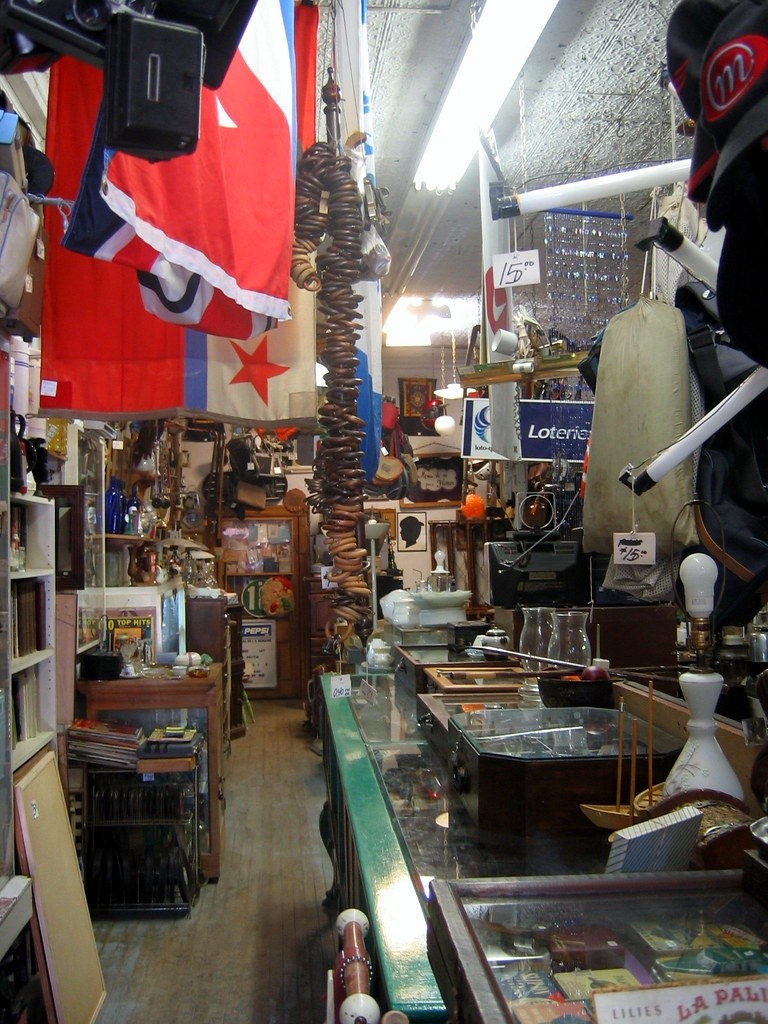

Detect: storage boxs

[395,644,519,700]
[544,920,627,968]
[447,707,688,831]
[625,916,768,972]
[650,946,768,983]
[447,621,490,651]
[552,968,644,1000]
[511,1001,594,1024]
[416,695,546,766]
[604,805,704,873]
[430,866,768,1024]
[424,665,537,695]
[495,970,566,1006]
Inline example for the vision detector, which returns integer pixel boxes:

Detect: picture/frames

[397,452,476,510]
[397,512,427,551]
[14,750,118,1024]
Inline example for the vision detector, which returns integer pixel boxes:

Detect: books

[0,501,156,769]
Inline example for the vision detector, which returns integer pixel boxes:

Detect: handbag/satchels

[210,429,290,514]
[0,170,40,318]
[106,13,203,158]
[575,289,767,629]
[360,417,419,500]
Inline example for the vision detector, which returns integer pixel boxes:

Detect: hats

[701,0,767,230]
[666,0,731,199]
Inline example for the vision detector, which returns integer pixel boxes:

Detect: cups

[173,666,186,676]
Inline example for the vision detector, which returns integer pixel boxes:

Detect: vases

[520,607,560,672]
[105,475,142,534]
[548,611,592,670]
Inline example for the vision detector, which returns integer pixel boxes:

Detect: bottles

[519,607,556,694]
[545,612,591,669]
[105,477,139,534]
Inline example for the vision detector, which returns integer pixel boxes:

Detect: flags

[33,0,385,486]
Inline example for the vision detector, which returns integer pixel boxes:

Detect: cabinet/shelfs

[78,663,225,882]
[189,599,247,740]
[209,504,312,698]
[11,492,61,977]
[302,574,339,673]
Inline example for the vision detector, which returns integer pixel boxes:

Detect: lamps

[434,337,456,436]
[430,549,451,575]
[413,0,560,193]
[661,553,746,802]
[433,330,476,400]
[364,520,391,629]
